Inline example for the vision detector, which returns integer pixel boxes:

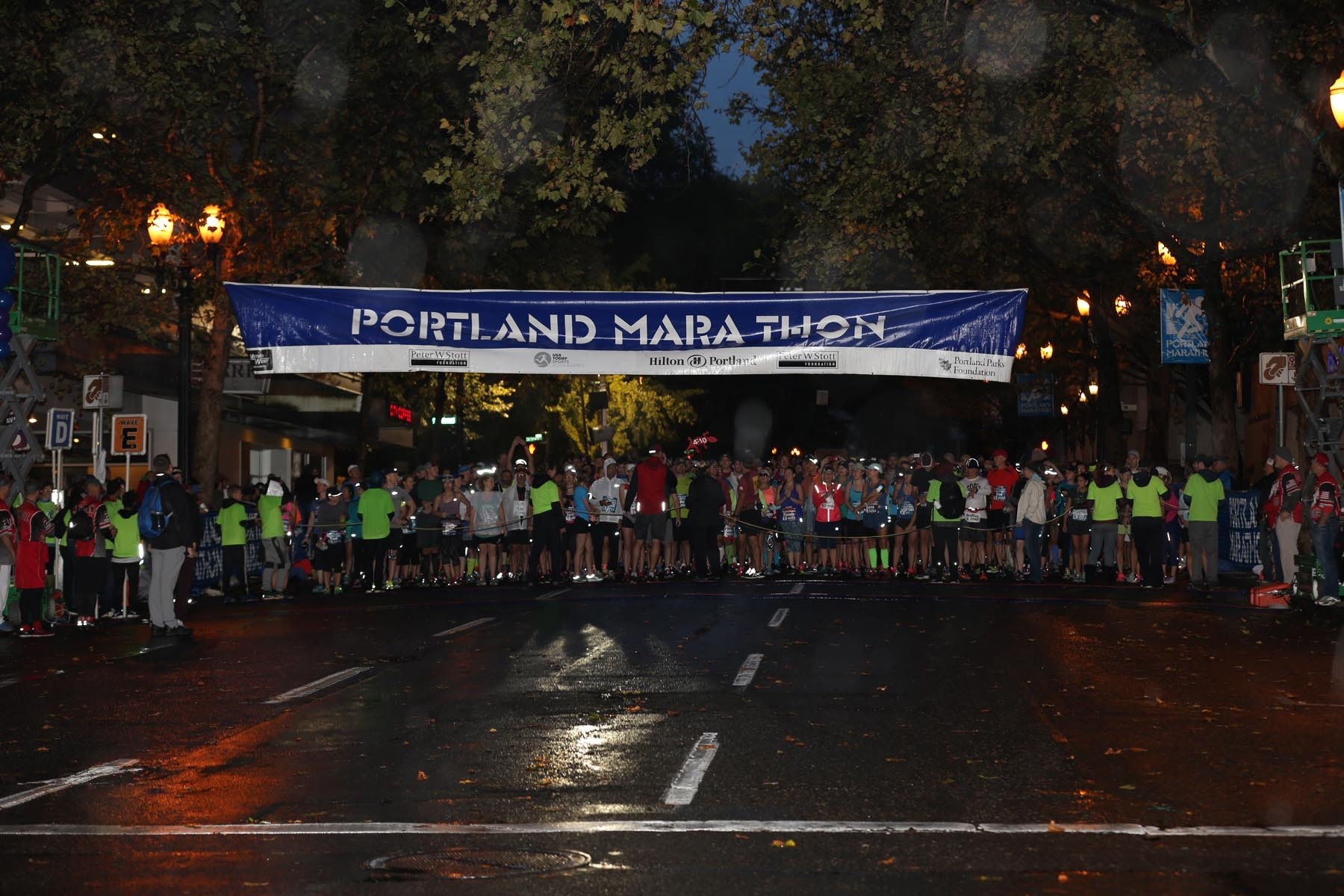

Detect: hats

[1314,452,1329,467]
[314,440,1227,496]
[1274,447,1292,462]
[154,453,169,473]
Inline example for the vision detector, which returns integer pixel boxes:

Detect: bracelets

[1123,514,1129,517]
[975,488,978,494]
[788,495,791,499]
[732,513,738,517]
[864,502,867,507]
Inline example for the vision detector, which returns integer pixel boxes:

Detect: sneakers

[1314,595,1340,606]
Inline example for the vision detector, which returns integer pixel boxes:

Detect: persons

[0,444,1344,638]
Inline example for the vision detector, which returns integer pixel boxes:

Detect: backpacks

[67,500,97,542]
[137,481,173,538]
[50,505,71,539]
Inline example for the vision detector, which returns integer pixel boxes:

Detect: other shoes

[151,624,192,638]
[0,621,15,631]
[18,621,43,636]
[67,607,141,618]
[76,620,100,631]
[187,588,294,604]
[314,561,1220,593]
